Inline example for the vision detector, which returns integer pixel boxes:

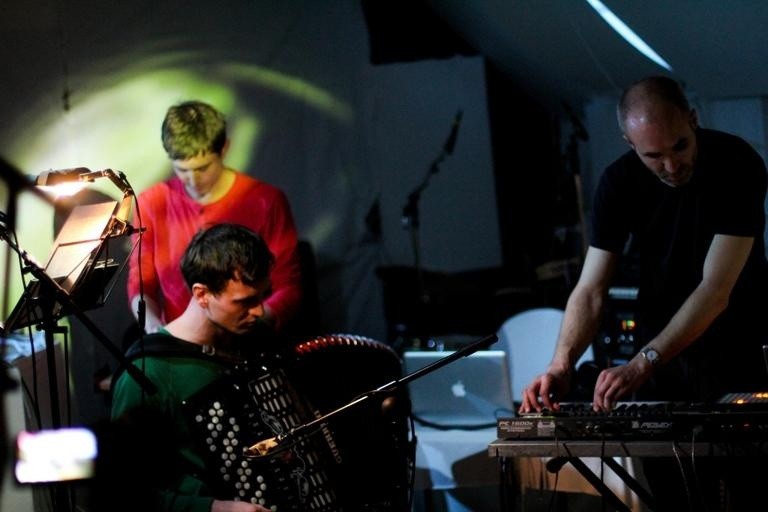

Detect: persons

[106,221,315,512]
[514,71,767,511]
[123,99,303,349]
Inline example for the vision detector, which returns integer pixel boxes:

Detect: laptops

[402,350,515,423]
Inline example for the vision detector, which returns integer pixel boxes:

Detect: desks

[488,437,726,512]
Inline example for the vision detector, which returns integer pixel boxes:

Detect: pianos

[497,390,768,443]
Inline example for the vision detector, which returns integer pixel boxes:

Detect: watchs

[640,343,665,367]
